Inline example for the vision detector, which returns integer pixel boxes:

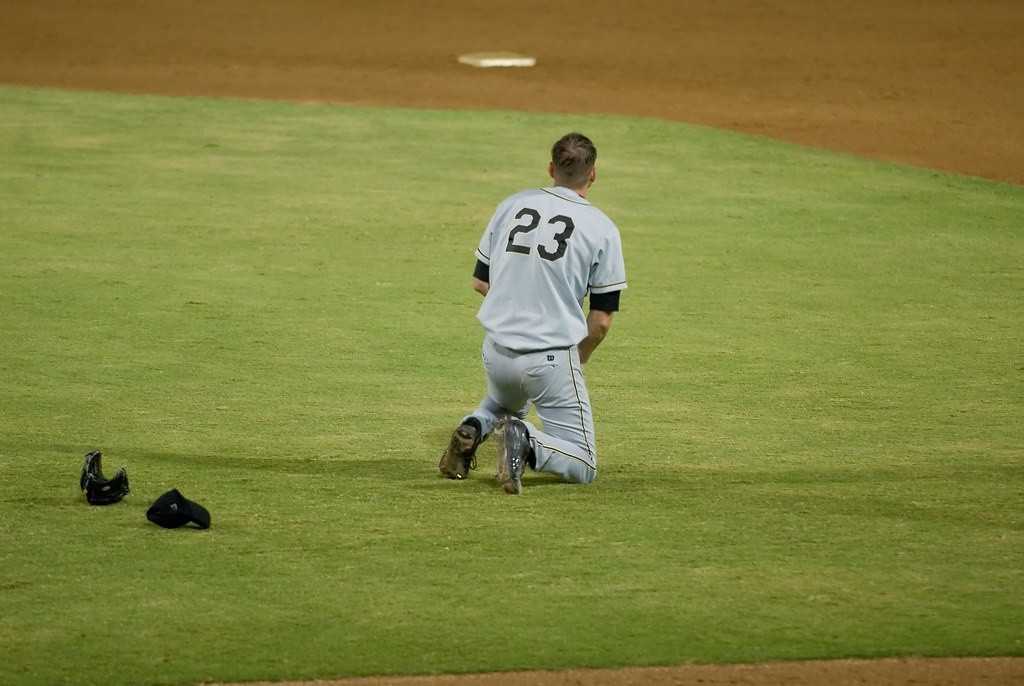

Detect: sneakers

[439,418,481,479]
[494,418,531,493]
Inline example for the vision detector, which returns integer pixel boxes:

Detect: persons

[440,132,626,492]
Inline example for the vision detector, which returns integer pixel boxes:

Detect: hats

[146,488,211,529]
